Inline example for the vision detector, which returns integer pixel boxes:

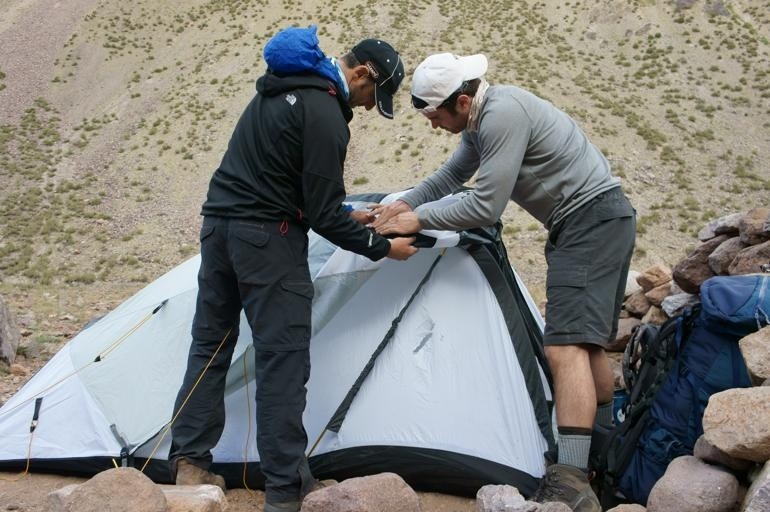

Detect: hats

[351,39,405,118]
[411,53,488,114]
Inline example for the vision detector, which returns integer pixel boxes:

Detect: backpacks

[589,274,770,512]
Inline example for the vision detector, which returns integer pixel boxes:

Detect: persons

[367,51,636,512]
[168,24,417,512]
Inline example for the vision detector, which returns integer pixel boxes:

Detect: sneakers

[175,459,227,494]
[526,463,603,512]
[264,479,339,512]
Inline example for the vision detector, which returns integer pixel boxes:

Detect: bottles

[611,385,631,427]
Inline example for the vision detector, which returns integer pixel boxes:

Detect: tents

[0,192,558,499]
